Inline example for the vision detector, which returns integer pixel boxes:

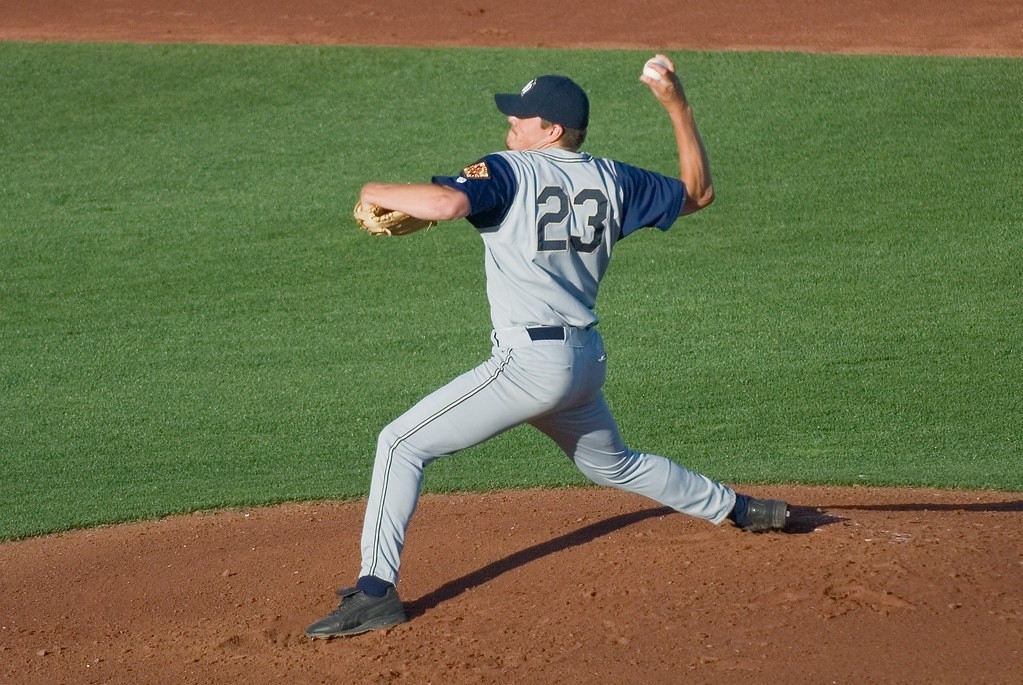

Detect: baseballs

[643,58,667,81]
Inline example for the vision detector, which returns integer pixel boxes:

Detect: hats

[494,75,589,128]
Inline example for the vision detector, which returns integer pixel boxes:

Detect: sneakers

[305,585,407,636]
[747,498,787,531]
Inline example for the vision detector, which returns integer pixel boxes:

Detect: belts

[494,327,589,347]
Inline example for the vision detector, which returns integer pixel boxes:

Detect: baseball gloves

[353,199,438,238]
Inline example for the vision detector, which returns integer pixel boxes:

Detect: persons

[306,52,790,637]
